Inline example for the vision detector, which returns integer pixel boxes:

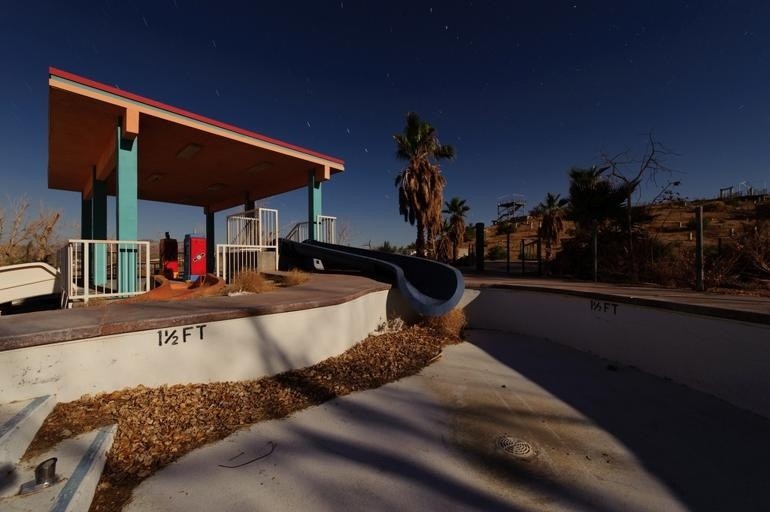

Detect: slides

[269,235,464,317]
